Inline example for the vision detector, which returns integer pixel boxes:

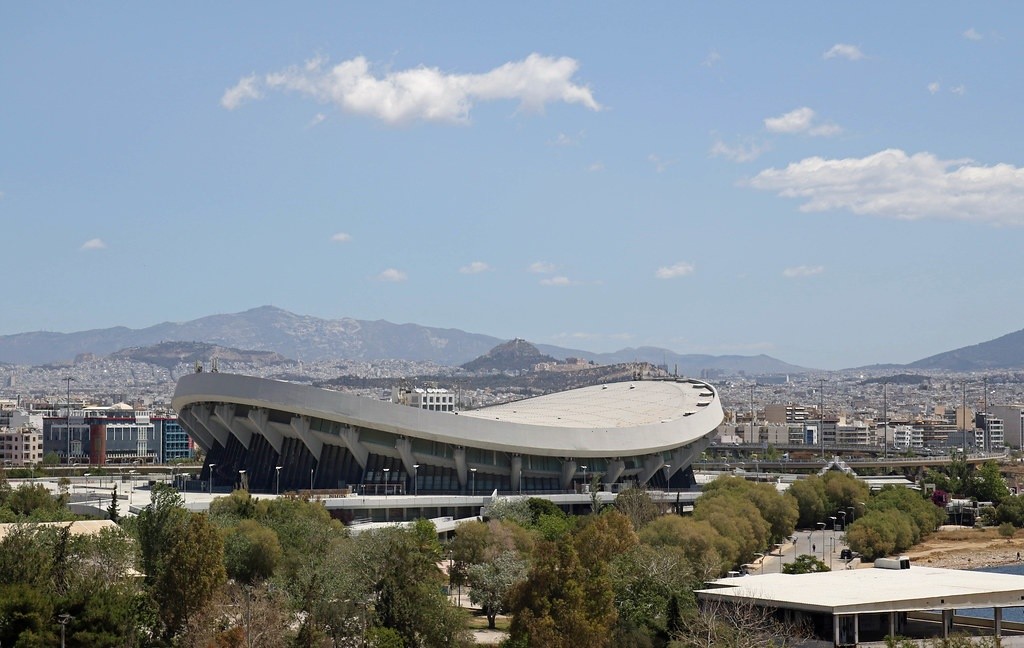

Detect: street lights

[518,468,525,498]
[469,466,477,495]
[383,467,389,495]
[181,471,189,505]
[72,462,78,490]
[275,465,280,495]
[665,462,676,490]
[413,463,419,496]
[581,464,588,492]
[127,470,134,506]
[30,464,35,484]
[85,472,89,505]
[309,468,314,493]
[754,501,866,575]
[206,464,214,497]
[239,468,247,491]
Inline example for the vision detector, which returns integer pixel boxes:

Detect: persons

[812,542,816,553]
